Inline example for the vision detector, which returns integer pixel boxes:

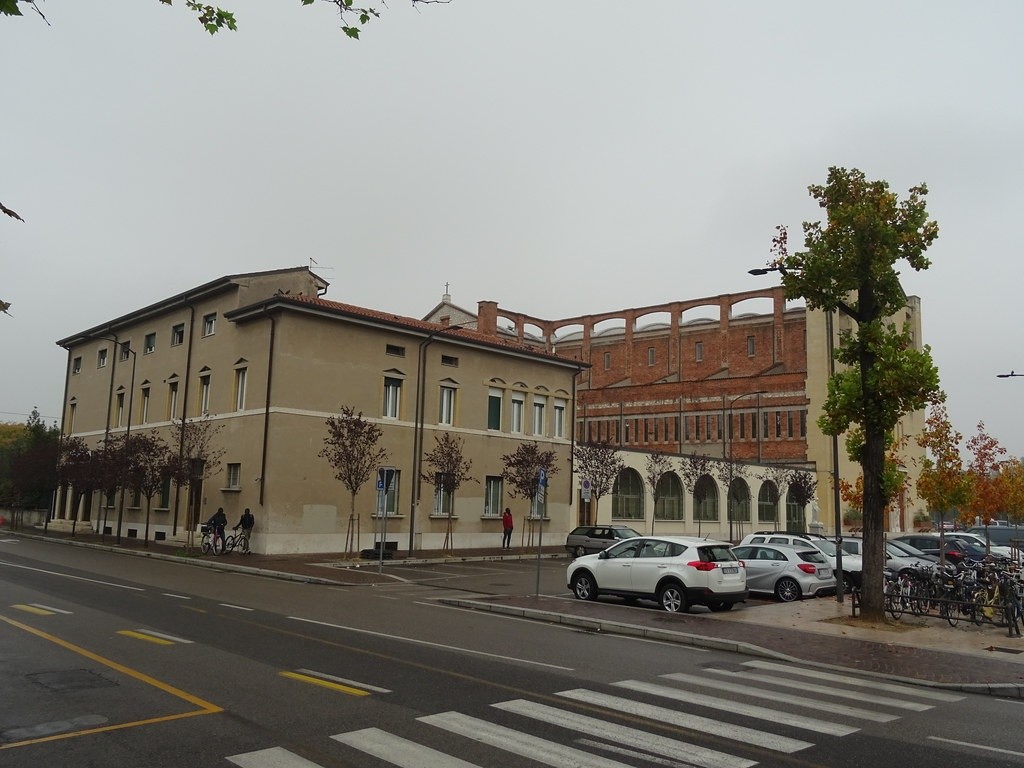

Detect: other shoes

[222,551,228,554]
[507,547,513,549]
[503,547,506,550]
[242,550,251,555]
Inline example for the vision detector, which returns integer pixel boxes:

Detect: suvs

[740,529,863,594]
[565,524,643,557]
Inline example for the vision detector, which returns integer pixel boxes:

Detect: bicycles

[200,522,225,557]
[849,552,1024,638]
[225,526,250,556]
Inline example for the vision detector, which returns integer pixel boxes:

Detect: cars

[893,535,987,573]
[938,514,1019,532]
[567,534,750,613]
[933,531,1024,566]
[827,537,958,584]
[730,542,838,603]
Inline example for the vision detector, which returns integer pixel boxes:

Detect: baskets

[918,567,934,580]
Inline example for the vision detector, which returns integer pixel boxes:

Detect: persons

[208,508,227,555]
[502,508,513,550]
[235,508,254,555]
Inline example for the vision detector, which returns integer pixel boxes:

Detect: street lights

[77,334,137,548]
[406,327,465,560]
[729,390,769,542]
